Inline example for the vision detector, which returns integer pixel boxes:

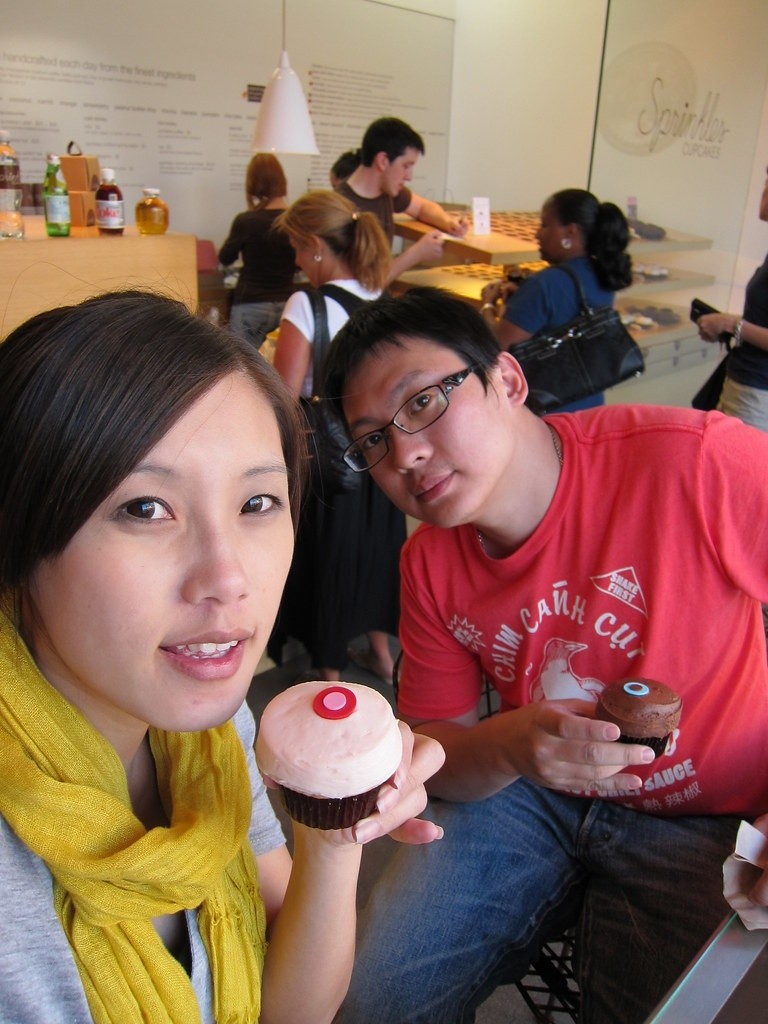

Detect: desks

[384,207,717,349]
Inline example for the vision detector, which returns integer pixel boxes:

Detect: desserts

[620,219,681,331]
[595,677,683,757]
[255,680,403,829]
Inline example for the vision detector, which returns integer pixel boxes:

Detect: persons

[698,165,768,431]
[267,189,409,683]
[330,147,362,191]
[331,285,768,1024]
[217,152,303,352]
[481,189,633,417]
[333,115,469,291]
[0,285,446,1024]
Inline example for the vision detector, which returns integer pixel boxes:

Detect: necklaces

[477,424,564,543]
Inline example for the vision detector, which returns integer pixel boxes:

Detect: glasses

[343,362,478,473]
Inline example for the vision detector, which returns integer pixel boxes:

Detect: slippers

[347,648,394,686]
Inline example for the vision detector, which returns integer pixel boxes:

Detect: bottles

[94,168,125,236]
[135,187,170,235]
[42,155,70,236]
[0,143,25,239]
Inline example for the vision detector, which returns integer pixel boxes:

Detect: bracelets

[735,318,745,347]
[480,303,498,316]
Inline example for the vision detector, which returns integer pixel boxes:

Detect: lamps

[249,0,320,155]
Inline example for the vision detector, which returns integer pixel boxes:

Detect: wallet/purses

[691,299,735,343]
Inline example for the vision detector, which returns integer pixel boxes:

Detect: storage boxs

[58,154,101,226]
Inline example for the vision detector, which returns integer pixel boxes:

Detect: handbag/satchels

[306,398,372,490]
[510,264,645,411]
[692,355,729,410]
